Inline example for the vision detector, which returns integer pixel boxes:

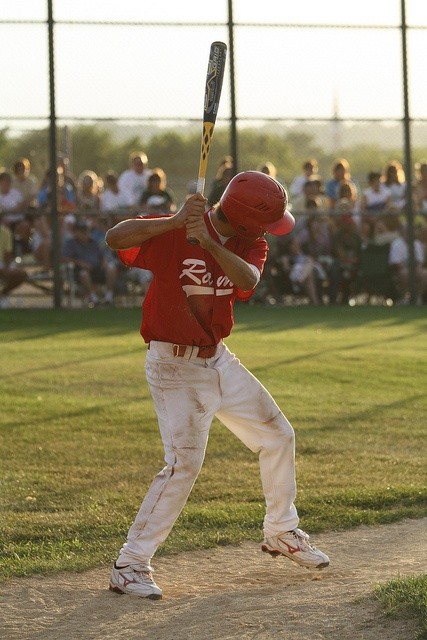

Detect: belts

[147,343,217,358]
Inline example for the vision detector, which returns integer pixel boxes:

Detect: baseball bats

[187,40,228,246]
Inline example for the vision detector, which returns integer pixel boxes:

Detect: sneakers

[108,562,163,600]
[262,527,330,569]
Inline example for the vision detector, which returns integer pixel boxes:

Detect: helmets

[219,170,295,236]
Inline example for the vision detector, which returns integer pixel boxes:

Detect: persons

[120,151,154,201]
[209,157,425,304]
[1,151,116,308]
[106,173,331,600]
[140,171,175,211]
